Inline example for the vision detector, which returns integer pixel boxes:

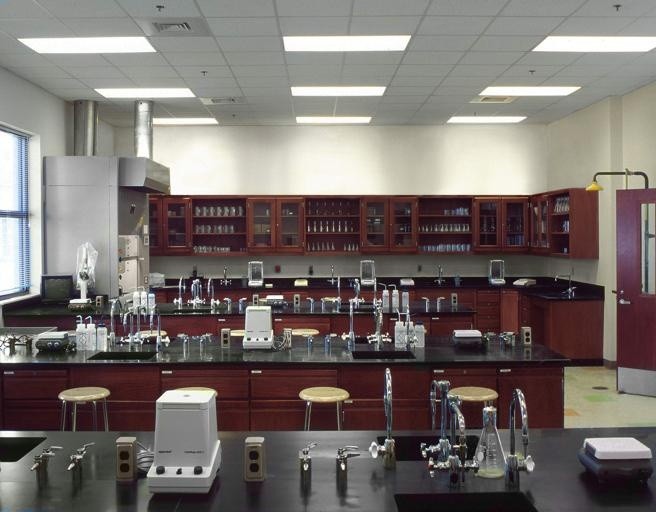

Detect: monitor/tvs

[40,275,73,306]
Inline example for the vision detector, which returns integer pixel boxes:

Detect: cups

[179,207,184,216]
[564,220,570,232]
[191,205,244,253]
[444,206,469,216]
[418,224,473,253]
[282,209,289,216]
[167,211,176,217]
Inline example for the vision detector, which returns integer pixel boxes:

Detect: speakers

[114,436,136,481]
[520,326,532,346]
[243,436,266,482]
[253,294,259,306]
[450,292,458,307]
[283,327,292,349]
[94,295,104,308]
[294,294,301,307]
[221,328,231,349]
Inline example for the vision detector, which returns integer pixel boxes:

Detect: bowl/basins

[135,329,168,343]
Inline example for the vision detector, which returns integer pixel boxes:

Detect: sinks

[173,309,219,313]
[87,351,157,360]
[377,435,493,463]
[118,337,176,345]
[339,308,377,313]
[352,351,416,359]
[0,437,47,462]
[394,492,537,512]
[341,302,374,306]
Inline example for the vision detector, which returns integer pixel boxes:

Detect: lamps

[586,172,655,292]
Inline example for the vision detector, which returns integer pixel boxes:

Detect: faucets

[137,306,147,333]
[349,302,357,346]
[223,266,228,281]
[150,307,162,336]
[179,275,186,299]
[207,277,215,300]
[504,389,530,490]
[123,311,134,337]
[384,368,393,440]
[438,264,443,285]
[110,299,122,333]
[554,274,571,288]
[447,394,466,485]
[430,378,449,439]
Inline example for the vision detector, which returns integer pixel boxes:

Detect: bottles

[473,405,509,480]
[306,200,360,251]
[379,282,390,307]
[148,293,156,309]
[554,196,571,213]
[388,284,399,309]
[138,285,149,310]
[393,308,426,349]
[132,287,141,308]
[402,290,410,309]
[76,314,107,352]
